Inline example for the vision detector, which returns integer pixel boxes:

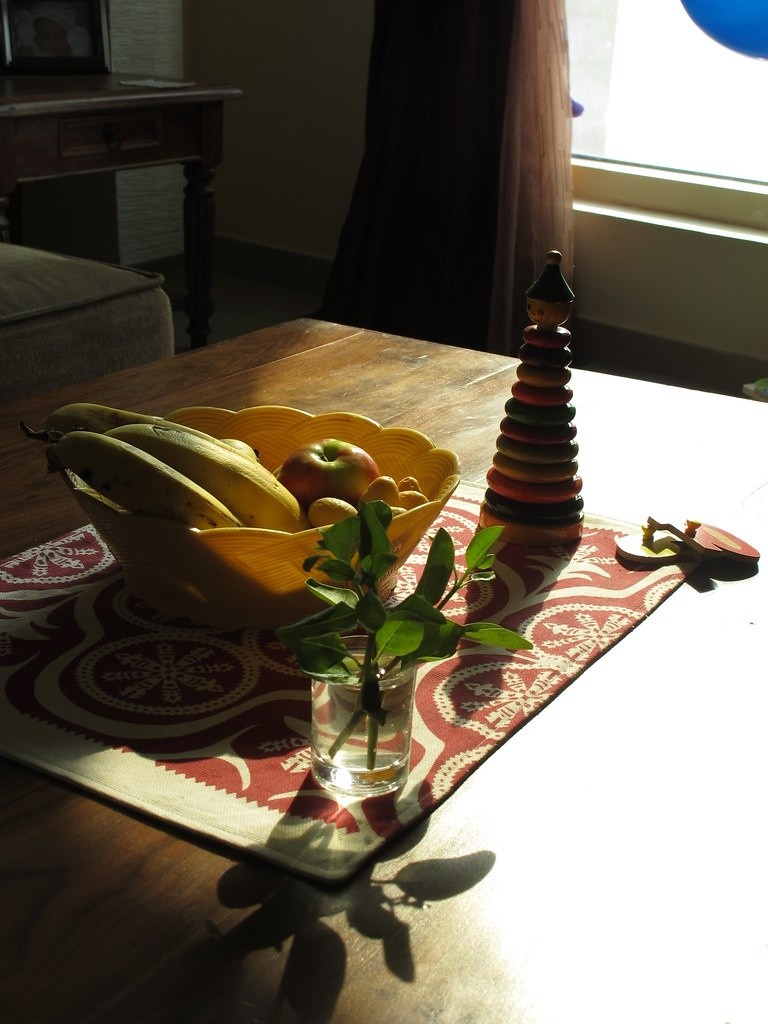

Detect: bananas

[25,409,309,555]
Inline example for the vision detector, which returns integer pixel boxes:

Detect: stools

[0,238,180,401]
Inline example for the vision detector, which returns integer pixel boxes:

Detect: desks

[0,72,244,352]
[0,311,767,1024]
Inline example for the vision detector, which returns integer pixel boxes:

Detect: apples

[276,437,381,506]
